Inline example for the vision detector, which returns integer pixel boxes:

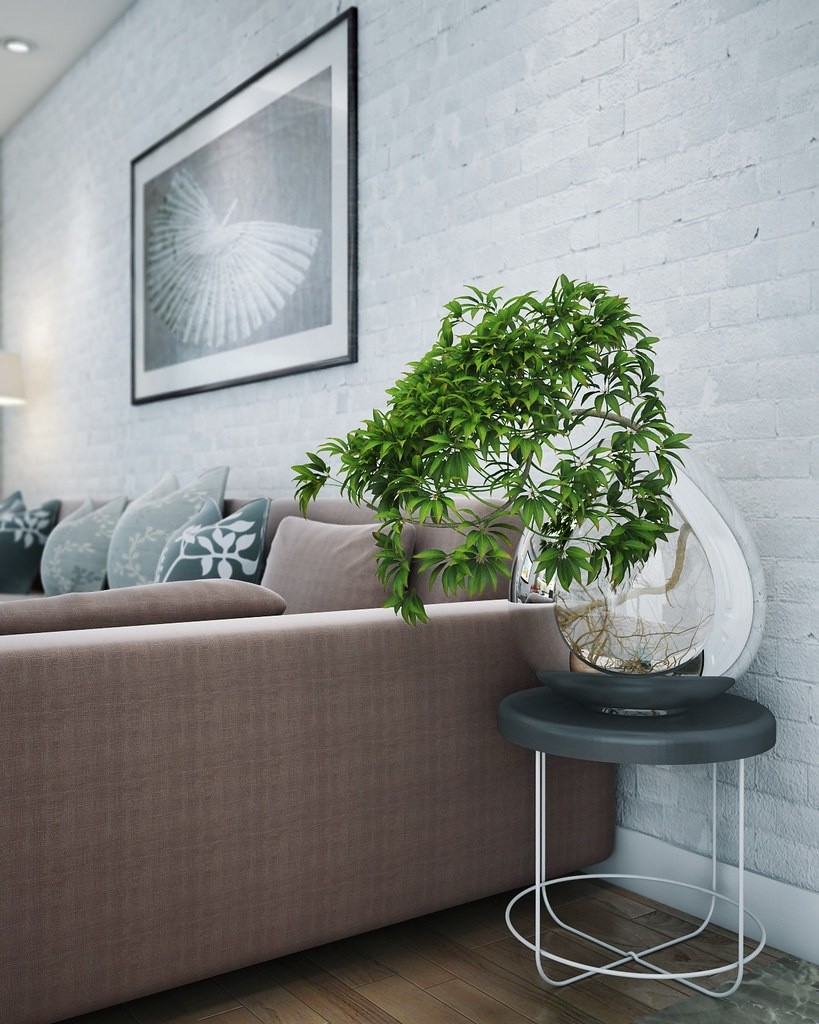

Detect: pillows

[40,497,127,598]
[153,498,272,585]
[0,492,61,596]
[105,466,230,589]
[258,516,415,615]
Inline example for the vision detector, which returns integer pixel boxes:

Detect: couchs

[0,495,616,1024]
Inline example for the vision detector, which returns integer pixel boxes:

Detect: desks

[498,685,776,997]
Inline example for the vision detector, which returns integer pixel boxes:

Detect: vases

[507,449,766,717]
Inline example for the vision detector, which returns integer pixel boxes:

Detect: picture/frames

[130,6,359,407]
